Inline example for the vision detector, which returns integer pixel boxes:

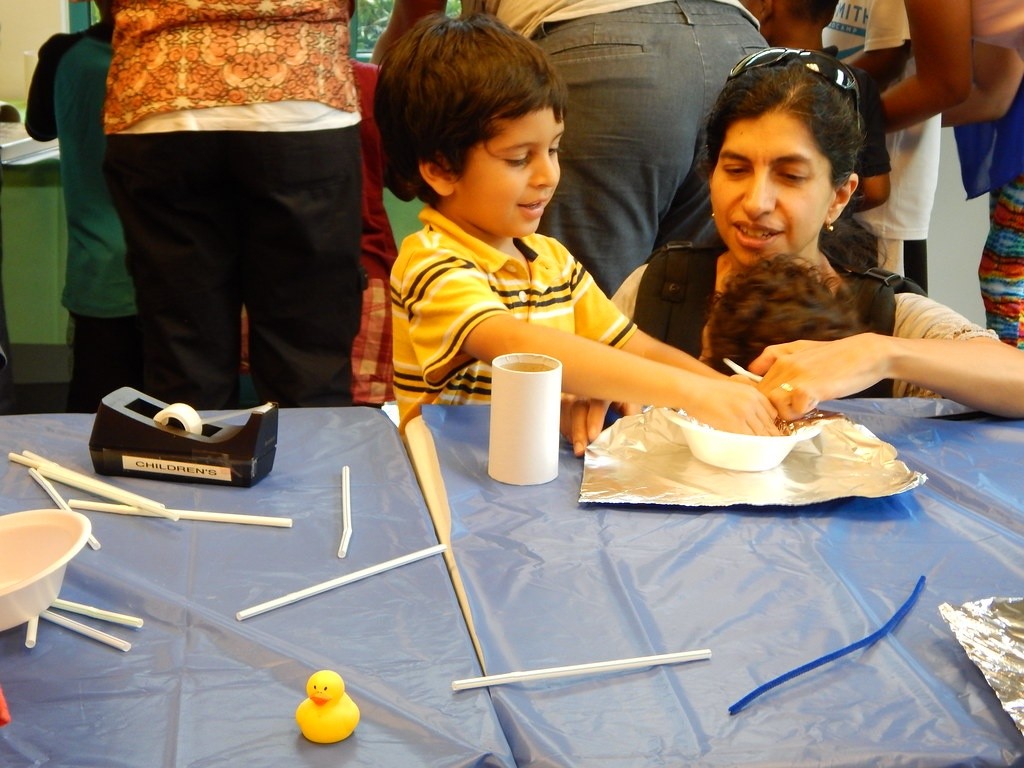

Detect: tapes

[153,403,202,436]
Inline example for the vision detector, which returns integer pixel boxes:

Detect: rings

[777,383,793,392]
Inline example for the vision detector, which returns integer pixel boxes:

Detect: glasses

[726,46,862,131]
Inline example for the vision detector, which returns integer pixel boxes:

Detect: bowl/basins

[0,509,93,633]
[658,406,823,474]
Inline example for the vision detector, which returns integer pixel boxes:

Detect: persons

[821,0,943,295]
[698,252,858,375]
[102,0,365,411]
[344,2,398,406]
[878,1,1024,347]
[561,46,1023,455]
[739,0,892,212]
[369,0,769,298]
[25,0,136,410]
[376,14,780,456]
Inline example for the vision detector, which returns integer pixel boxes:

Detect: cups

[486,352,563,486]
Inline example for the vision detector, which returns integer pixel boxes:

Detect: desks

[1,396,517,768]
[402,397,1023,768]
